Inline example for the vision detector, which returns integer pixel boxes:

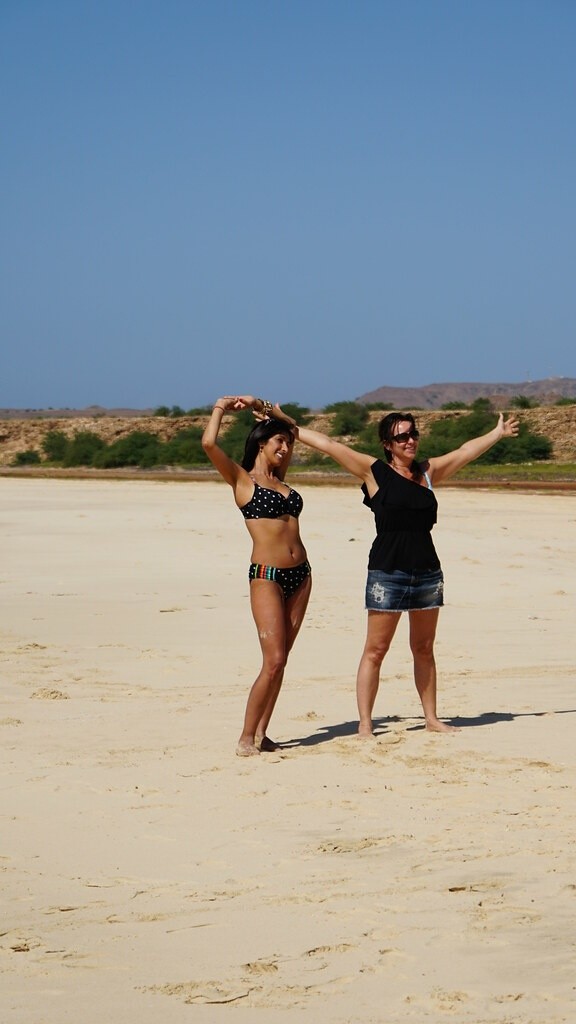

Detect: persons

[252,402,520,736]
[202,396,312,757]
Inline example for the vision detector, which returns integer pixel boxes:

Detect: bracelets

[253,399,273,416]
[213,406,225,415]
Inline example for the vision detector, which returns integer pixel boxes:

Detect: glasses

[390,431,419,443]
